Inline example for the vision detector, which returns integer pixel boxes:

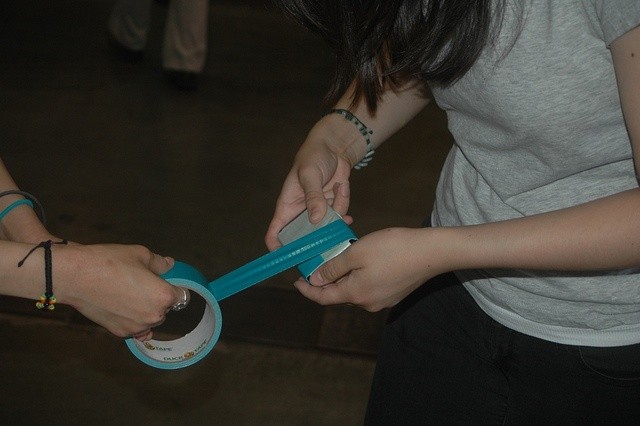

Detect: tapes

[125,220,361,369]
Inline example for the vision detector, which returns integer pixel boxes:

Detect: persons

[104,0,208,94]
[264,0,639,426]
[0,158,191,337]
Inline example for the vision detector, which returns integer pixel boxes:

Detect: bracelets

[0,199,33,218]
[18,240,68,311]
[321,110,375,170]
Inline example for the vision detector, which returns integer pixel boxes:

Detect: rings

[170,287,191,312]
